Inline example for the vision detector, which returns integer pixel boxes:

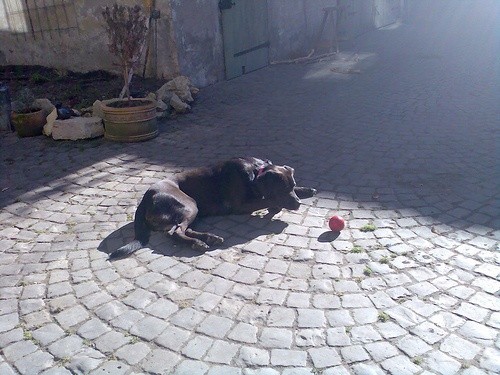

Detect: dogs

[107,156,318,260]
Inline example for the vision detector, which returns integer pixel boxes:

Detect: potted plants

[11,87,47,139]
[99,3,161,142]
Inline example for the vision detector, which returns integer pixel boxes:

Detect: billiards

[330,216,344,232]
